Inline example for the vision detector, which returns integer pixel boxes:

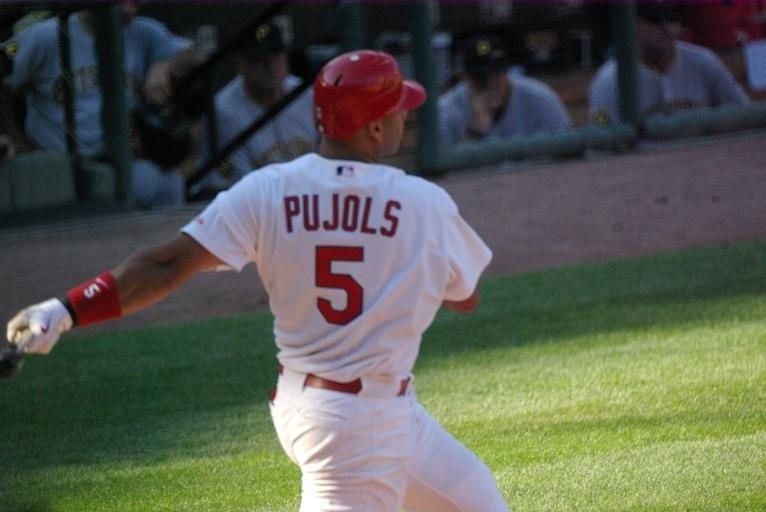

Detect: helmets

[314,50,427,141]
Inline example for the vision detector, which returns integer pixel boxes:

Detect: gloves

[6,295,76,355]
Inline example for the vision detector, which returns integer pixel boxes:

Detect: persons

[5,51,507,512]
[1,1,762,210]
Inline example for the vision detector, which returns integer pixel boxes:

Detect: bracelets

[67,268,125,327]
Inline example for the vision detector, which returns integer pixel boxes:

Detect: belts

[278,364,410,396]
